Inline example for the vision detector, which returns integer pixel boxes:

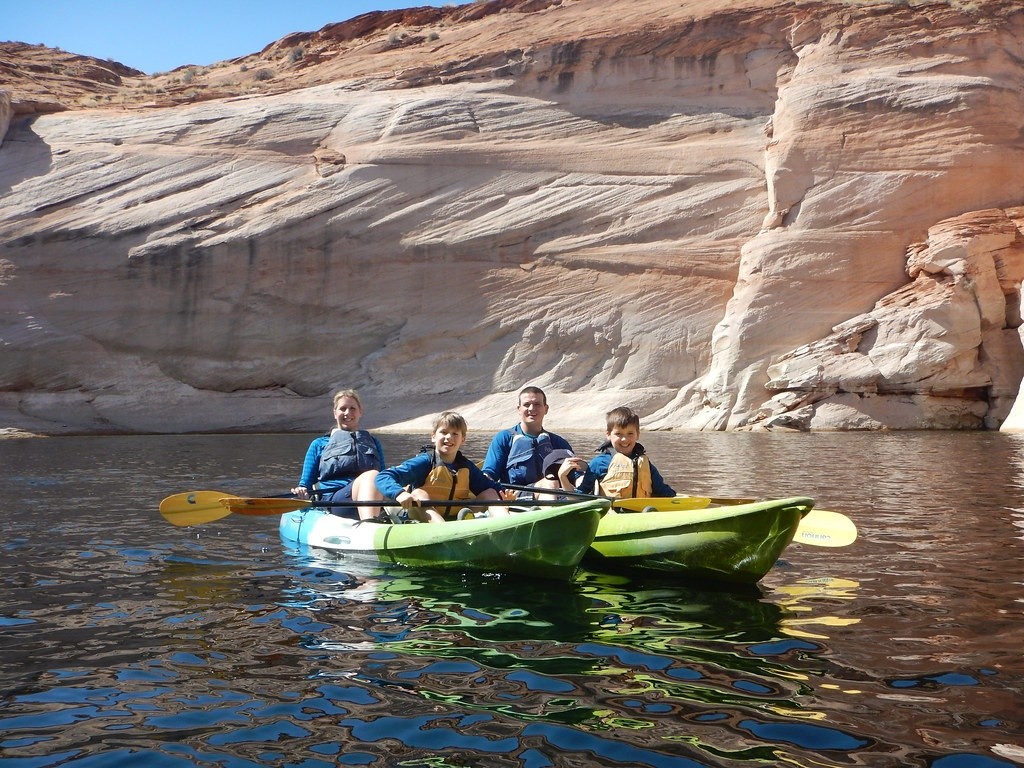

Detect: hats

[542,449,588,480]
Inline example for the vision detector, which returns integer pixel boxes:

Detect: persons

[290,389,421,524]
[373,411,542,523]
[481,386,585,509]
[558,406,677,513]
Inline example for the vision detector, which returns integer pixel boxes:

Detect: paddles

[711,493,858,549]
[156,486,346,528]
[216,495,712,517]
[498,478,578,496]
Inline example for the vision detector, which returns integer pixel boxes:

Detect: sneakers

[457,508,475,520]
[643,507,658,512]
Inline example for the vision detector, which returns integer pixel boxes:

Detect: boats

[278,495,613,582]
[584,494,817,592]
